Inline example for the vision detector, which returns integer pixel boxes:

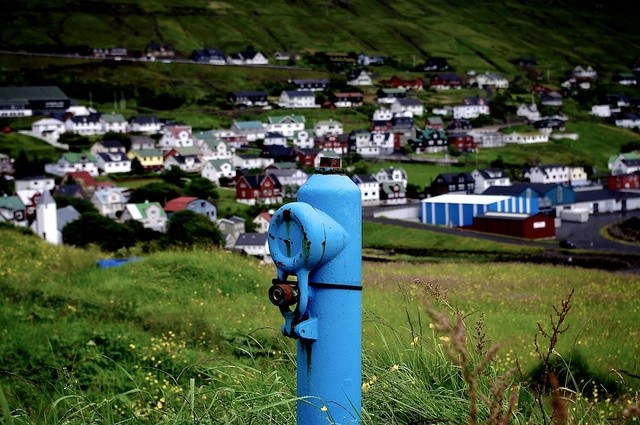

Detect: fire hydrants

[264,156,362,425]
